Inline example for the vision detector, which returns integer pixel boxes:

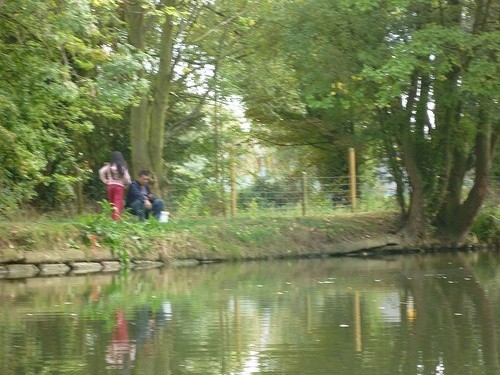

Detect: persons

[99,151,131,221]
[126,171,165,225]
